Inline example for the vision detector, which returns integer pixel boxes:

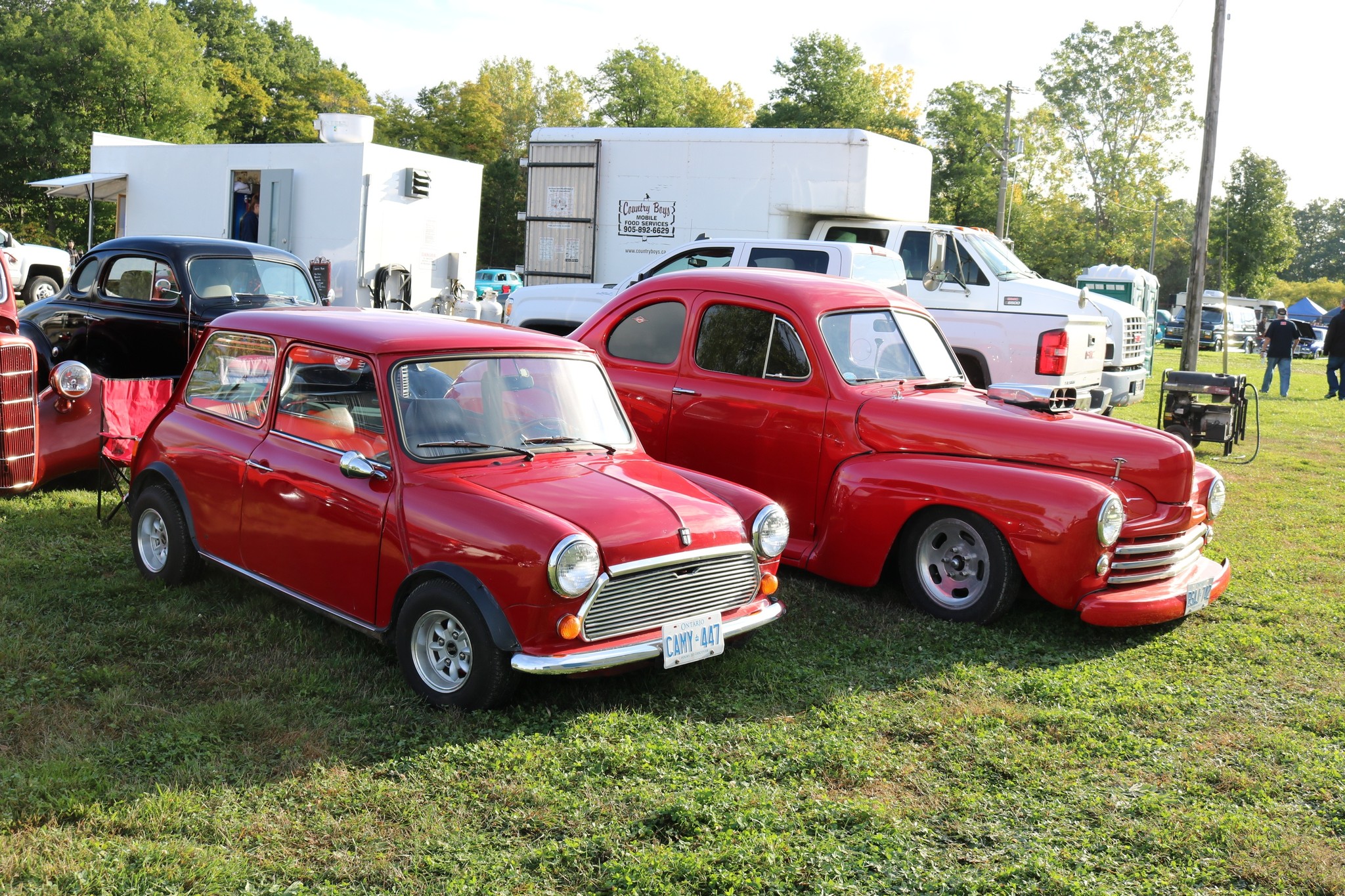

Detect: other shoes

[1324,392,1336,399]
[1339,397,1345,401]
[1259,390,1268,395]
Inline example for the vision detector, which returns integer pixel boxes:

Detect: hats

[1277,308,1287,315]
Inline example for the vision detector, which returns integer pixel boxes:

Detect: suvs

[0,246,106,497]
[0,228,72,306]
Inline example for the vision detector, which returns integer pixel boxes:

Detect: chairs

[196,270,232,298]
[404,398,468,458]
[97,378,173,522]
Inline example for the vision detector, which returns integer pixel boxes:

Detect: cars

[1260,319,1328,360]
[122,306,788,712]
[475,269,524,300]
[441,266,1231,629]
[19,235,325,394]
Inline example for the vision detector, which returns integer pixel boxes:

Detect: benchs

[215,391,369,428]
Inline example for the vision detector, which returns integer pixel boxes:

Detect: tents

[1285,297,1341,324]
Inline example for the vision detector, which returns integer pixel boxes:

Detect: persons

[1322,298,1345,402]
[63,238,83,273]
[239,191,260,243]
[1255,316,1267,335]
[1258,308,1302,396]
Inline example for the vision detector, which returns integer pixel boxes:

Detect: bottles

[1261,351,1268,363]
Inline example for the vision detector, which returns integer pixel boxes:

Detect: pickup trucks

[505,239,1111,416]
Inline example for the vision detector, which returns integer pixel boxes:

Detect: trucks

[1171,289,1289,337]
[515,127,1145,407]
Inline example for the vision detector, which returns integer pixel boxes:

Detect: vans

[1158,304,1259,354]
